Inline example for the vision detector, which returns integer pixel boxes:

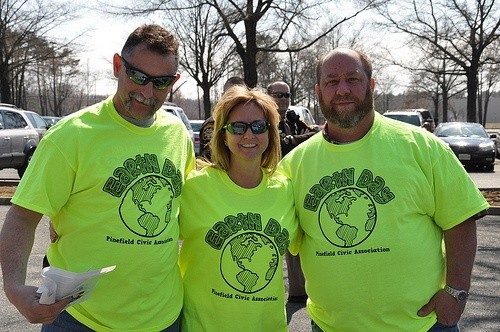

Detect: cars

[382,109,500,173]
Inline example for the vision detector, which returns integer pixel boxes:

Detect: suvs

[1,103,320,179]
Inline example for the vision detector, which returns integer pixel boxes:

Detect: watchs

[444,286,469,303]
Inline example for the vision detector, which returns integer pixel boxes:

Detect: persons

[278,48,491,332]
[0,24,193,332]
[47,88,304,332]
[198,77,318,162]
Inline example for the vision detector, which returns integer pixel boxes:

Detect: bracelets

[287,295,304,302]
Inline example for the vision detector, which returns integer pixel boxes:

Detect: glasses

[270,91,291,98]
[120,55,176,91]
[223,120,271,136]
